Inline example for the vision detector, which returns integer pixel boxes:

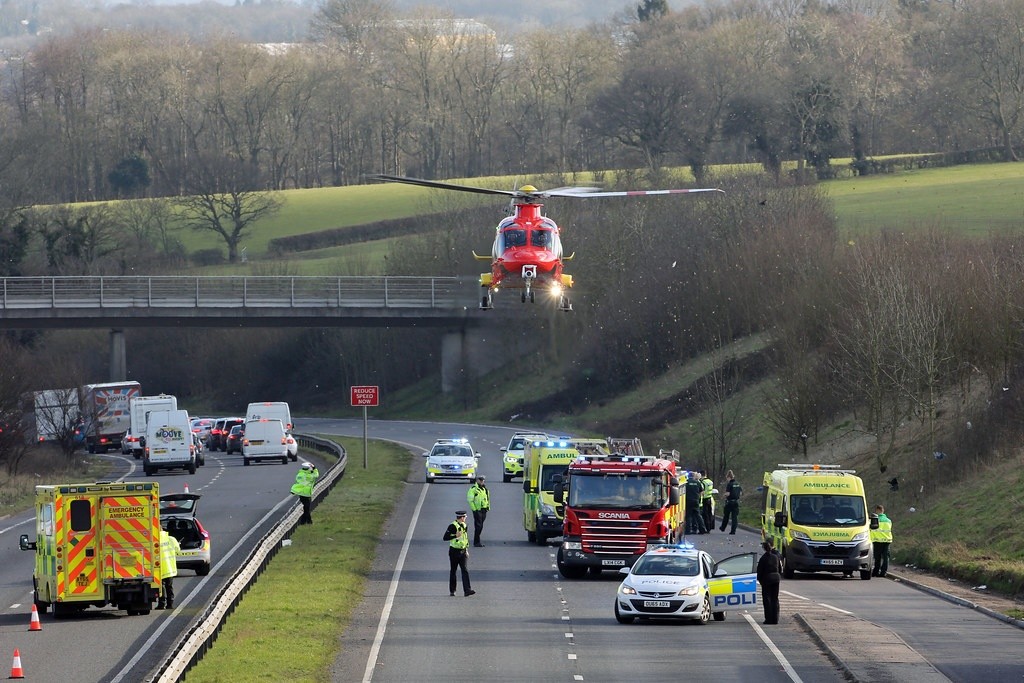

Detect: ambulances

[760,462,880,580]
[19,481,164,620]
[522,437,611,546]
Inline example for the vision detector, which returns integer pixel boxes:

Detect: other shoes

[464,590,476,597]
[728,532,735,534]
[449,591,456,596]
[474,543,485,547]
[719,526,725,531]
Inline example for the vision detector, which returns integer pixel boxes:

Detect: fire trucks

[551,452,690,581]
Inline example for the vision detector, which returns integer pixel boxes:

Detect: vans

[239,417,291,466]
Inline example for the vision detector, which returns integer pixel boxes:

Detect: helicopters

[357,173,725,313]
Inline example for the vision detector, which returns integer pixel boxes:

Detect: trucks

[139,409,197,476]
[241,401,295,436]
[127,394,178,459]
[0,380,143,454]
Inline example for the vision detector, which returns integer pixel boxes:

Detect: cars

[191,431,205,468]
[286,434,299,462]
[499,431,550,483]
[421,438,482,485]
[119,426,133,455]
[614,543,759,625]
[188,415,245,456]
[158,492,211,576]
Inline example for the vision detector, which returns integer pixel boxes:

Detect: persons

[459,448,468,456]
[686,470,719,535]
[719,470,742,535]
[539,233,547,242]
[467,474,491,547]
[290,463,320,525]
[758,538,783,625]
[155,524,181,609]
[442,511,476,597]
[869,504,893,578]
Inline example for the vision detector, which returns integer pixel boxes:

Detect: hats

[475,476,486,480]
[301,462,313,468]
[455,510,467,517]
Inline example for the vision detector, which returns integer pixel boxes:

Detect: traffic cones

[166,490,177,508]
[184,482,190,501]
[8,648,25,679]
[27,603,43,631]
